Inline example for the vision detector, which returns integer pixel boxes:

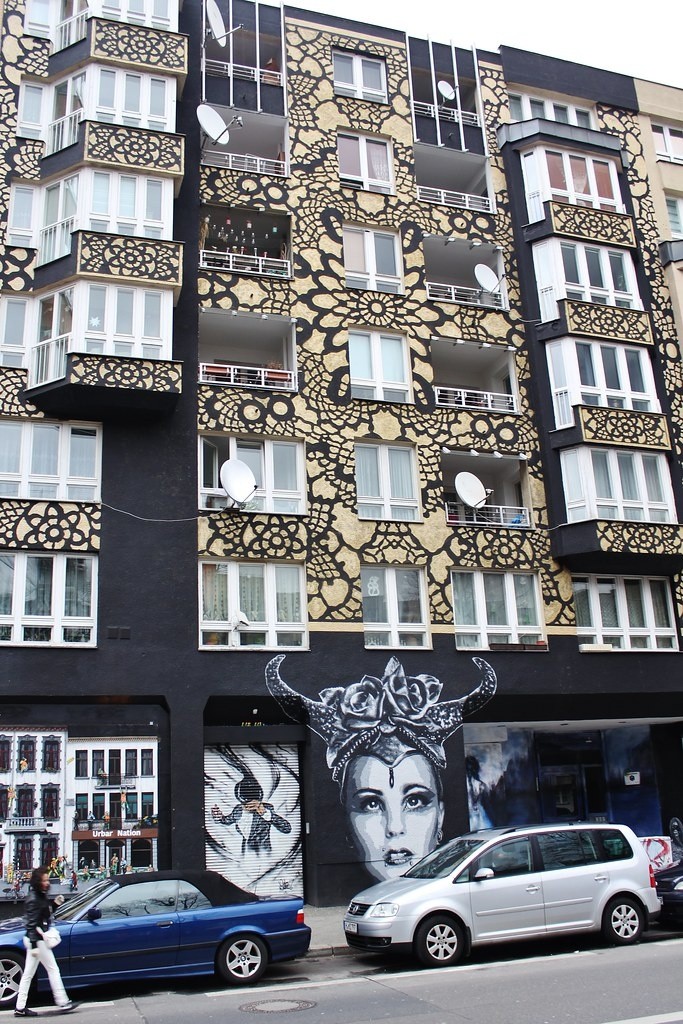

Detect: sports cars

[0,868,313,1009]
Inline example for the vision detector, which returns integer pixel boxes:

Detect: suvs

[340,819,664,971]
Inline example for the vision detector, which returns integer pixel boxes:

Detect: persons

[15,870,30,886]
[80,857,107,882]
[7,862,13,884]
[14,878,20,900]
[110,853,127,875]
[148,864,153,871]
[72,810,80,830]
[69,867,78,890]
[14,867,83,1017]
[102,811,110,831]
[87,811,96,830]
[48,855,69,877]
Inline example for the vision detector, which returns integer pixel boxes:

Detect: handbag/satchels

[43,928,62,949]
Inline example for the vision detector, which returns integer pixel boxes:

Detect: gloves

[32,948,40,957]
[54,895,65,906]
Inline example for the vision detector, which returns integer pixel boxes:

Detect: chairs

[520,845,528,859]
[502,843,515,860]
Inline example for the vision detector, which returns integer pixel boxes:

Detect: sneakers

[60,1002,80,1012]
[14,1007,37,1017]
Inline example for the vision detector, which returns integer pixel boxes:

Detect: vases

[206,366,227,375]
[264,371,289,380]
[489,643,525,650]
[537,641,544,644]
[525,644,547,650]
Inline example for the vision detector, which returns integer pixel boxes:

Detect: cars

[653,854,683,935]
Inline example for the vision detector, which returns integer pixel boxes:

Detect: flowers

[265,362,283,369]
